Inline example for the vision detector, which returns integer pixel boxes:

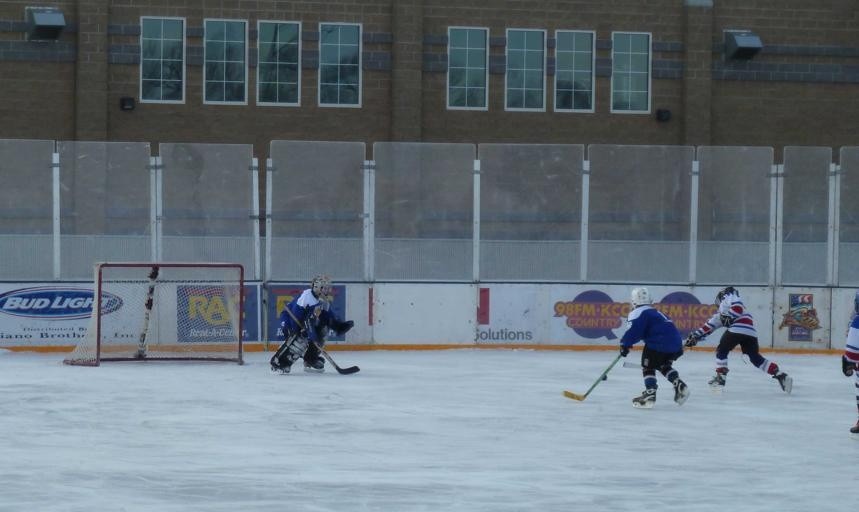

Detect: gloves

[686,334,699,347]
[720,314,736,328]
[619,343,629,356]
[840,355,856,377]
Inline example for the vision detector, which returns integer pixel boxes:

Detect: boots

[330,318,354,336]
[772,372,787,391]
[673,378,687,401]
[632,385,657,404]
[708,372,728,385]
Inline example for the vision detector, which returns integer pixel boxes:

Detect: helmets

[311,273,331,297]
[630,287,651,307]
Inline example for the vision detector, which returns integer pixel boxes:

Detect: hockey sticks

[562,353,622,401]
[623,322,722,369]
[281,301,359,374]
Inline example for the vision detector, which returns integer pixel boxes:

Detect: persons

[685,286,792,394]
[620,288,690,408]
[842,291,859,432]
[270,274,355,375]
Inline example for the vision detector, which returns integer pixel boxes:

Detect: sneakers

[281,366,290,373]
[304,360,324,370]
[851,420,859,434]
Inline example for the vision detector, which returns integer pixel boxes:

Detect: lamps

[122,98,135,111]
[657,109,671,122]
